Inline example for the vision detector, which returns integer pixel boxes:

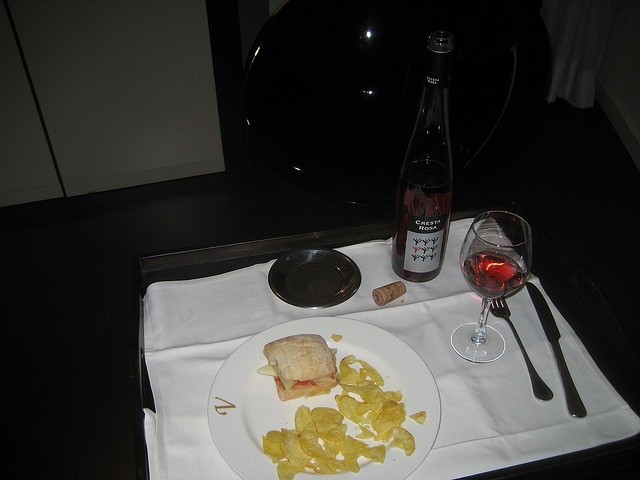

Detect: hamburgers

[258,334,338,401]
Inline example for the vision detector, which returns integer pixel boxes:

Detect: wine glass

[451,210,532,363]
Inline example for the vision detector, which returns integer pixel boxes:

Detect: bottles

[391,31,454,283]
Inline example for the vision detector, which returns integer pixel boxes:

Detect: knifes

[525,282,586,417]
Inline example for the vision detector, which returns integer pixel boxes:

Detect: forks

[490,299,554,402]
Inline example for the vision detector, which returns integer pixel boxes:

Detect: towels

[143,218,640,479]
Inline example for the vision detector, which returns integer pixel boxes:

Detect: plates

[207,316,442,480]
[268,248,362,308]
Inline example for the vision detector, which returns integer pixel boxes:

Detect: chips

[262,355,426,480]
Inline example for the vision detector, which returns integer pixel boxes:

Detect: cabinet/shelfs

[0,2,226,208]
